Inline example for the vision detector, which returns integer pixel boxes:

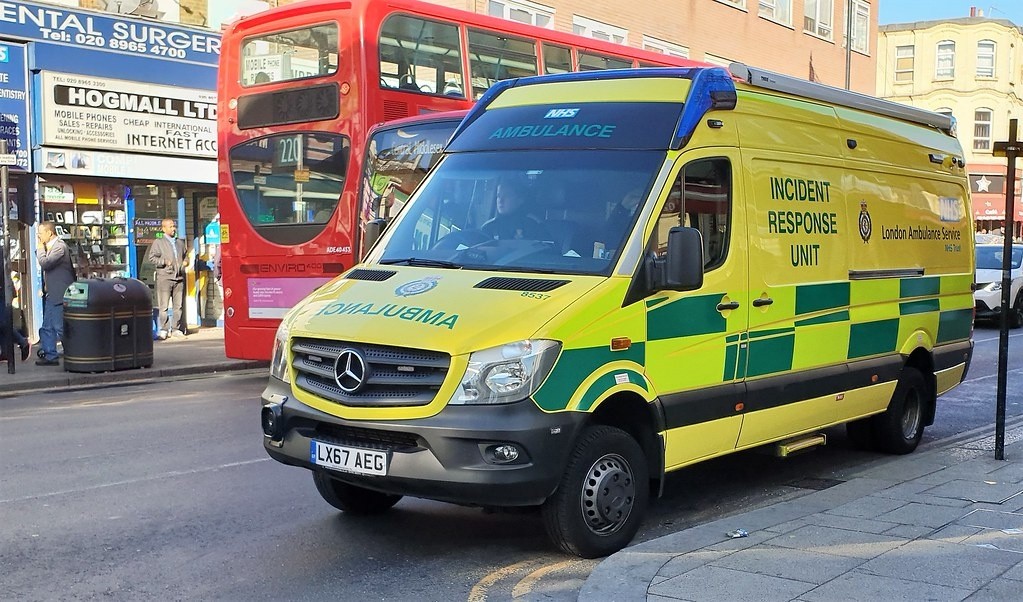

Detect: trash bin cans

[61,276,154,373]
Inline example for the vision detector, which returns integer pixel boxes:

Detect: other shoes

[171,329,183,337]
[35,358,59,366]
[157,330,168,340]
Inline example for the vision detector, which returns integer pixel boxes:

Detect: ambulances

[262,61,976,560]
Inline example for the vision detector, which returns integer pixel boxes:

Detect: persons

[481,182,540,242]
[35,221,77,366]
[606,191,643,245]
[0,225,31,363]
[976,228,987,235]
[148,218,190,339]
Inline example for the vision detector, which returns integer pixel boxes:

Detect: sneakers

[21,343,31,361]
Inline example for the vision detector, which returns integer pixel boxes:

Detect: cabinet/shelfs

[39,184,129,280]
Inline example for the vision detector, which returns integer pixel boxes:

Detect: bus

[217,1,739,359]
[352,107,546,262]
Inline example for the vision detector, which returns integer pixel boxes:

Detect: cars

[974,242,1023,332]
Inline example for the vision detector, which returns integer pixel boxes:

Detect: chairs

[534,175,589,251]
[398,75,420,91]
[442,82,462,97]
